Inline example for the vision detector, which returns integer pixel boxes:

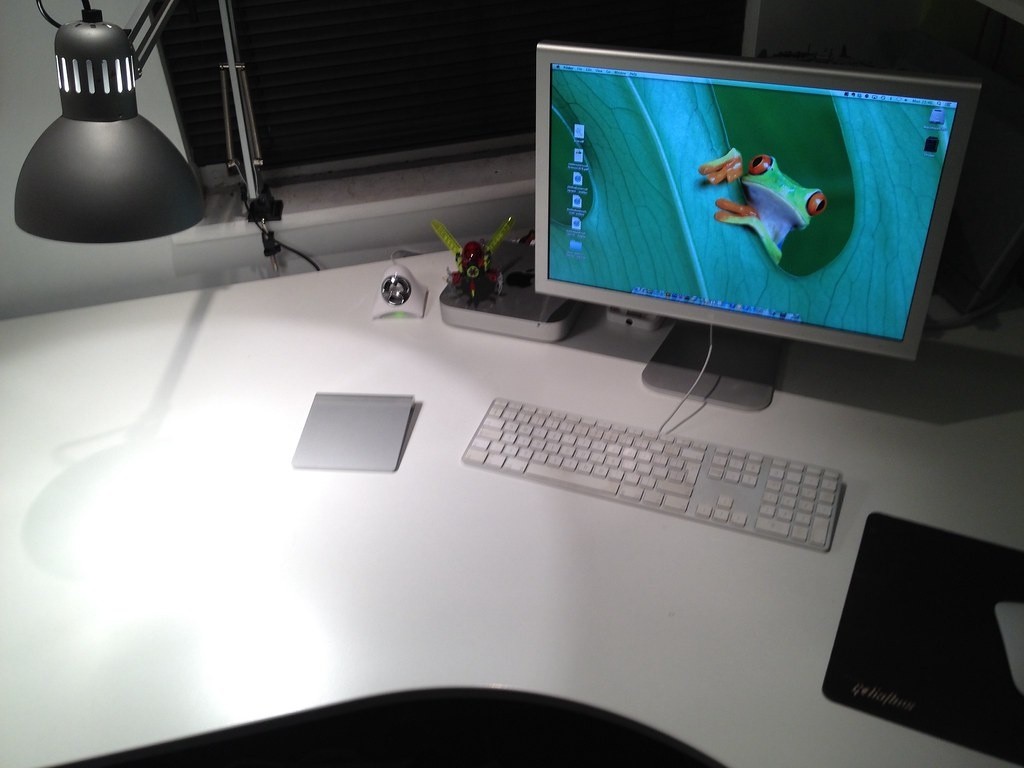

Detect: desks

[0,233,1024,768]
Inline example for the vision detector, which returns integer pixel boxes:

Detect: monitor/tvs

[531,41,983,414]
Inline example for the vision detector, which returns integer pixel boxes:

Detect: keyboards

[459,397,844,554]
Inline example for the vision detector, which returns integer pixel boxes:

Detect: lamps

[14,0,321,274]
